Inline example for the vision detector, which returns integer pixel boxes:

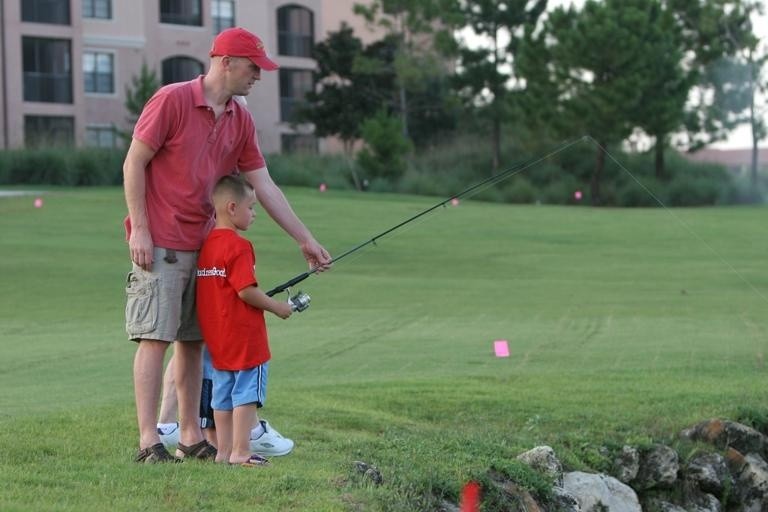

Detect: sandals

[174,438,219,464]
[134,441,185,465]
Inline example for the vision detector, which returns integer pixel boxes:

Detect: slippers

[241,453,273,466]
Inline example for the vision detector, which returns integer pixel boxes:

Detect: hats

[208,27,282,72]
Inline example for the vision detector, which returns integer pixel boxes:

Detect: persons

[156,93,293,456]
[119,26,334,465]
[194,175,294,465]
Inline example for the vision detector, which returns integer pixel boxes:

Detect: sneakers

[248,418,296,457]
[155,421,181,446]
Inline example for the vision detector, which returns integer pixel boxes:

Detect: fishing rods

[265,136,591,298]
